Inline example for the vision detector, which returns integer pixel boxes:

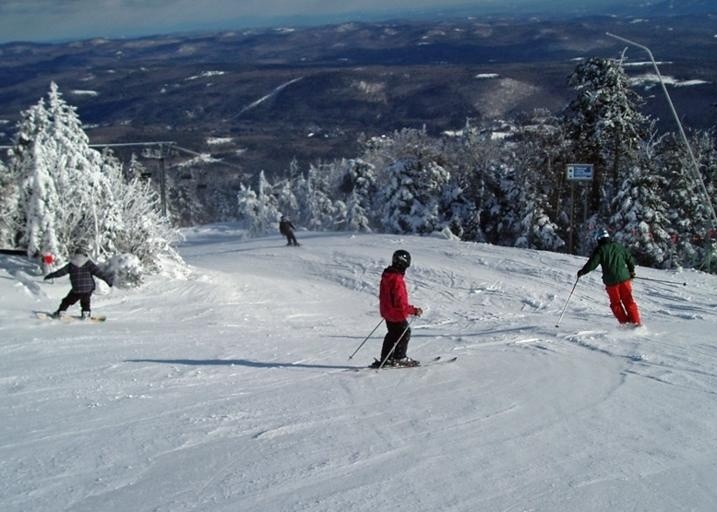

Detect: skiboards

[357,356,458,373]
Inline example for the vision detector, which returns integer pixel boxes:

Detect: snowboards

[36,311,107,323]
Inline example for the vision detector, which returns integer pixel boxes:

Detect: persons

[578,227,641,326]
[378,250,421,367]
[279,215,297,247]
[44,247,112,320]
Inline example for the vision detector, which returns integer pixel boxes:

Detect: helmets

[392,249,411,273]
[595,227,611,244]
[75,248,88,258]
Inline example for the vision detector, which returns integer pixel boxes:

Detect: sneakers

[81,311,91,322]
[52,310,66,320]
[380,356,418,367]
